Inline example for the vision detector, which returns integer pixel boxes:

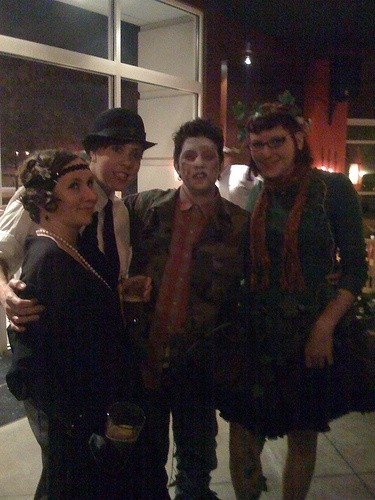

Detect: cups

[104,402,146,462]
[122,269,146,329]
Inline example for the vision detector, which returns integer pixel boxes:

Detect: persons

[6,148,152,500]
[217,102,375,500]
[0,108,157,500]
[119,116,252,500]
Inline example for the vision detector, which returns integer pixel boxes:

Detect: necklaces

[32,229,112,292]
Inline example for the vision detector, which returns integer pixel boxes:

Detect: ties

[103,200,120,283]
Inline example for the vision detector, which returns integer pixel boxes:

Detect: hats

[82,107,157,154]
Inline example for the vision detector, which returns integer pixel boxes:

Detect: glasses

[247,132,296,152]
